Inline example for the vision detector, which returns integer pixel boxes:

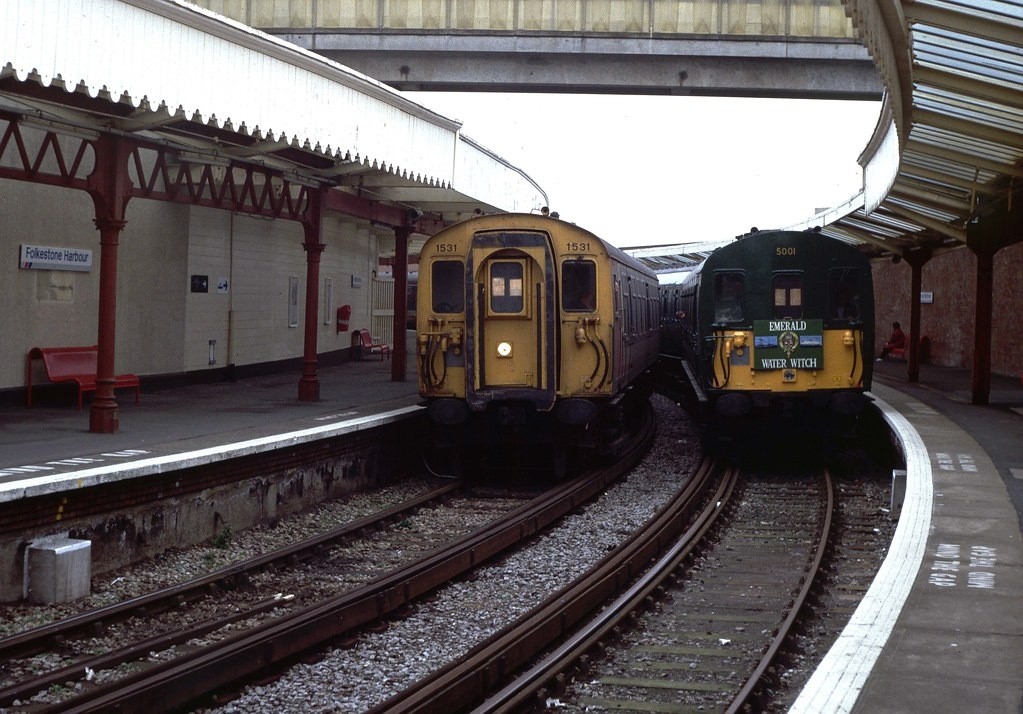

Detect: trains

[660,226,866,393]
[418,212,656,445]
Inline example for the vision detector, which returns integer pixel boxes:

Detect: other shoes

[876,358,883,362]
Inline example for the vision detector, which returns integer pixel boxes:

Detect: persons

[876,322,905,362]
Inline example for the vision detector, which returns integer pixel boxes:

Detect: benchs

[884,334,929,364]
[27,345,139,411]
[354,329,389,362]
